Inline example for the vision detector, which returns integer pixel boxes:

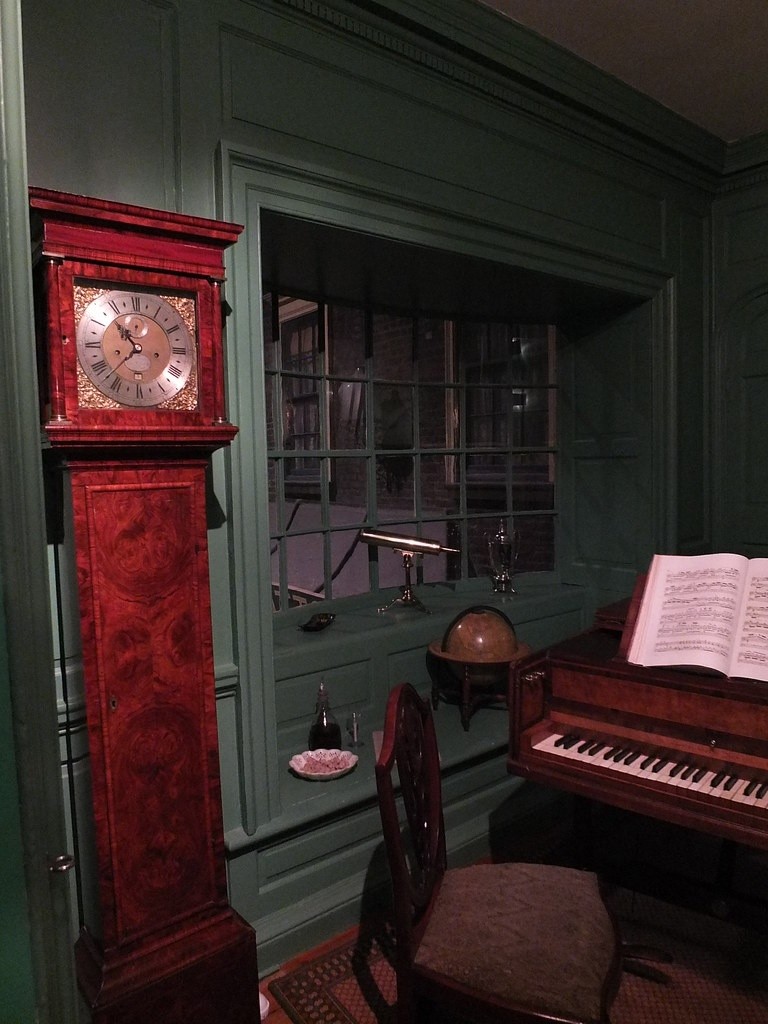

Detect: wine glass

[348,713,366,747]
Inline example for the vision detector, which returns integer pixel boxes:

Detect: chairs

[374,681,622,1024]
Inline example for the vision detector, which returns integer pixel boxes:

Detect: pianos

[506,592,768,935]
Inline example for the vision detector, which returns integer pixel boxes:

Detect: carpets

[268,817,768,1023]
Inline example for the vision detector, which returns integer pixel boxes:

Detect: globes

[426,605,532,732]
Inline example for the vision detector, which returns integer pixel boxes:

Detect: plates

[289,748,358,780]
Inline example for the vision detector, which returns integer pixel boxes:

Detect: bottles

[308,691,341,751]
[483,518,521,595]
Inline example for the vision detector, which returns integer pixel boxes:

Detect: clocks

[23,185,261,1023]
